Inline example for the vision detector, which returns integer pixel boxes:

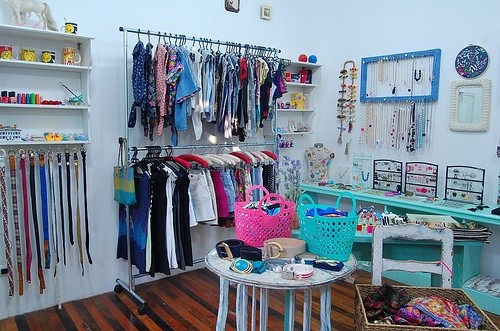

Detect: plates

[282,263,314,280]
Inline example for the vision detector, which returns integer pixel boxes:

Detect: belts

[0,148,93,299]
[215,238,314,280]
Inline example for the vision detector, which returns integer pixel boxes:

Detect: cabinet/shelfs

[0,23,93,146]
[265,61,325,136]
[300,181,500,315]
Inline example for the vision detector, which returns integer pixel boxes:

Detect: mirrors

[450,79,490,132]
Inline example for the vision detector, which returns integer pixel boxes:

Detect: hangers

[125,141,274,176]
[136,28,279,61]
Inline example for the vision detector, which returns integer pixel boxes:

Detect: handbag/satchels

[297,192,358,261]
[234,186,295,248]
[113,139,136,206]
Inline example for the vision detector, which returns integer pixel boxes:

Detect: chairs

[373,224,454,289]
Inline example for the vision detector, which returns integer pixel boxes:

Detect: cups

[0,45,18,60]
[21,47,36,62]
[60,22,78,34]
[62,47,82,65]
[41,50,57,64]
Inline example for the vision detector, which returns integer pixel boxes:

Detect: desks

[204,246,356,331]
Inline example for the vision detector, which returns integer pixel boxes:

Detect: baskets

[354,283,499,331]
